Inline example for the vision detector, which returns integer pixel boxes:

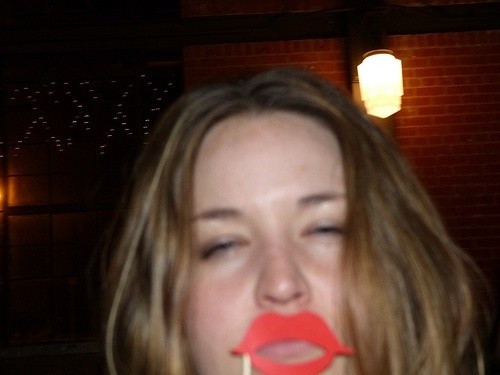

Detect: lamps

[356,47,404,121]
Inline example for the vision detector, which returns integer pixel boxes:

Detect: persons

[89,62,491,375]
